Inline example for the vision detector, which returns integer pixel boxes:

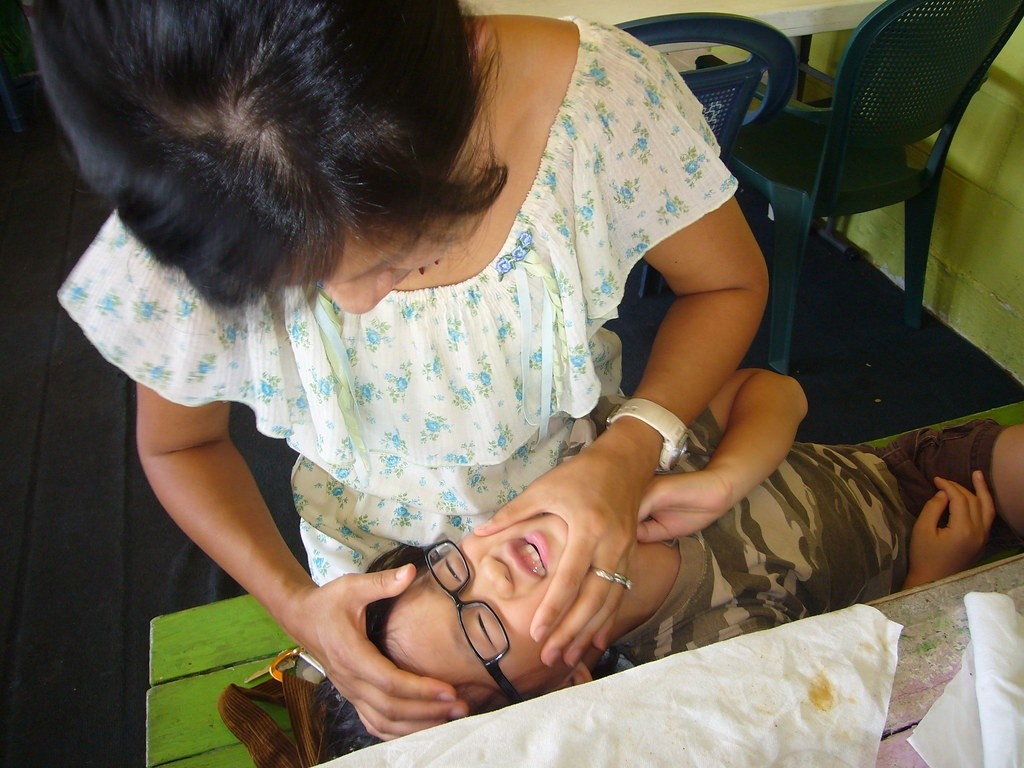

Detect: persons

[22,0,770,745]
[314,363,1024,721]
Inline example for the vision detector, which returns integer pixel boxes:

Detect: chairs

[605,13,804,306]
[730,0,1024,379]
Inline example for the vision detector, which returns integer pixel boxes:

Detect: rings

[614,572,632,590]
[592,567,613,581]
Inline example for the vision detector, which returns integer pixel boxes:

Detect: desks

[464,1,885,101]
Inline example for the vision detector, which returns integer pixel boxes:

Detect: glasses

[422,540,526,704]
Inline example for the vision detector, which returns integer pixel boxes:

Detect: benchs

[709,30,1023,388]
[145,396,1024,768]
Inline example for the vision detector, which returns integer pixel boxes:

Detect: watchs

[606,398,688,474]
